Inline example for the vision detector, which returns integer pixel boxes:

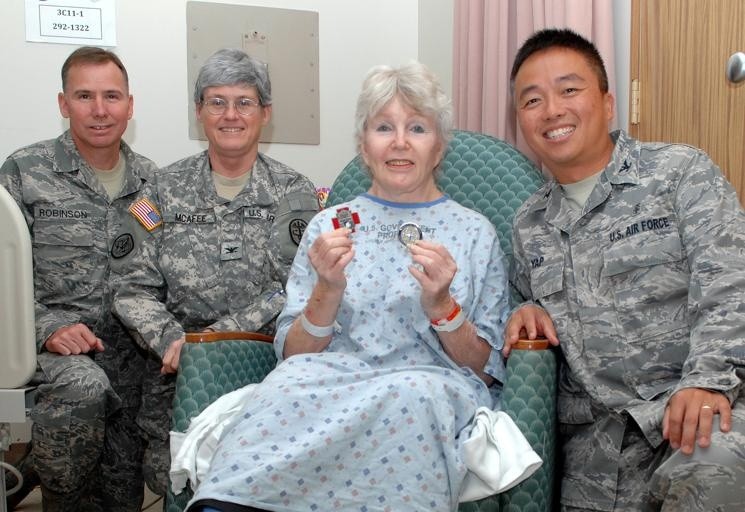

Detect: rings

[702,405,711,409]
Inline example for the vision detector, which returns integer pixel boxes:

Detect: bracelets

[298,311,342,338]
[430,297,465,333]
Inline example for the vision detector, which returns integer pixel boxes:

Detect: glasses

[202,96,258,116]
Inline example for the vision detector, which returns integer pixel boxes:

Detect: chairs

[167,127,558,512]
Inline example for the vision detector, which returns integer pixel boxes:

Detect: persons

[183,59,509,512]
[107,49,322,512]
[0,46,160,512]
[501,29,745,512]
